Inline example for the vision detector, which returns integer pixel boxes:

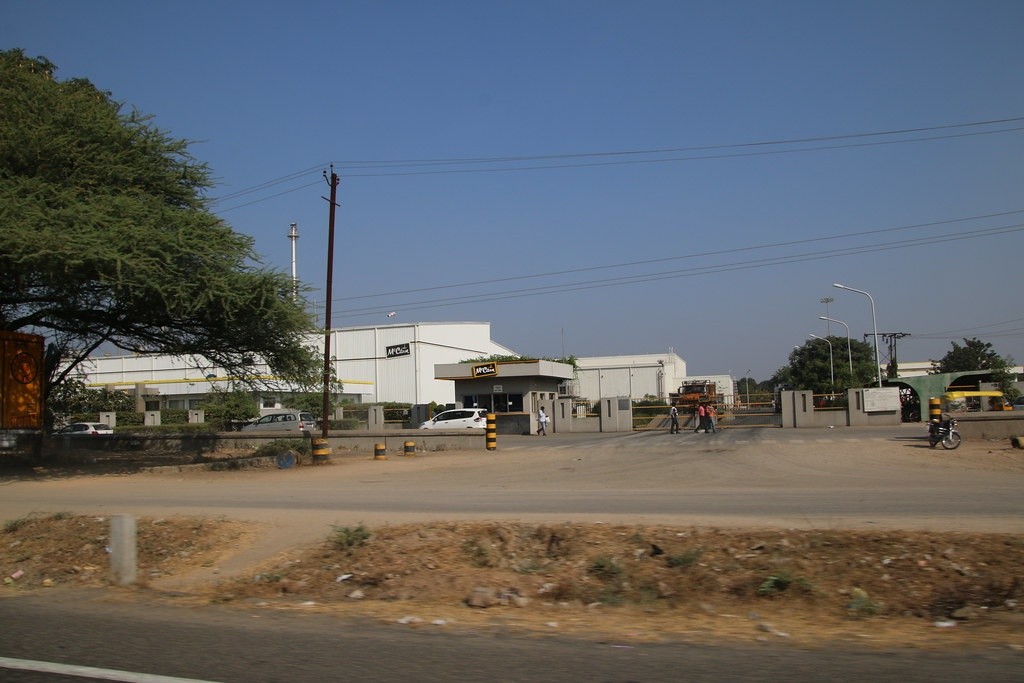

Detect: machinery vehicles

[669,378,726,422]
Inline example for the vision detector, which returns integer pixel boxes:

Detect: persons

[670,401,681,434]
[537,406,548,436]
[694,401,718,434]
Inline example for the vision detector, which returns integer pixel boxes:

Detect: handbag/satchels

[546,416,550,423]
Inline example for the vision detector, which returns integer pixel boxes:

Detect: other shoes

[537,431,540,435]
[671,432,674,434]
[543,433,547,436]
[694,431,699,433]
[676,432,680,434]
[705,431,709,433]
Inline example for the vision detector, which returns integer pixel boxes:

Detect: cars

[50,421,114,437]
[1012,395,1024,411]
[240,411,316,432]
[418,408,487,430]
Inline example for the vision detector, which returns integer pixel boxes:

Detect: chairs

[281,416,286,421]
[274,418,278,422]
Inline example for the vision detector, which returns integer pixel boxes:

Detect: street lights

[809,334,834,386]
[745,369,751,409]
[832,283,881,386]
[820,316,853,374]
[287,221,300,303]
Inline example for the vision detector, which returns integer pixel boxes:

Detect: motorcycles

[940,391,1015,413]
[927,413,962,450]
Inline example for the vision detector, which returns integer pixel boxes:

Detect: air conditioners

[557,384,574,398]
